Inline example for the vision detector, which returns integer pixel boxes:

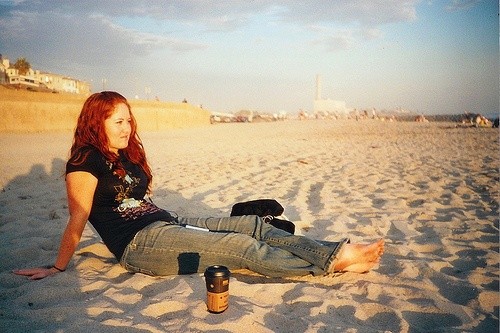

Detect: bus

[9,75,49,88]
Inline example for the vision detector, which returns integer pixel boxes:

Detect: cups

[204,265,230,314]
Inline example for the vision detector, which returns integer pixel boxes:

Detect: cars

[211,111,240,122]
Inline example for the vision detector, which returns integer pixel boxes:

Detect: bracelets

[53,265,67,272]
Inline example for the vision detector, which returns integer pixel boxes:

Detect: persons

[12,92,385,280]
